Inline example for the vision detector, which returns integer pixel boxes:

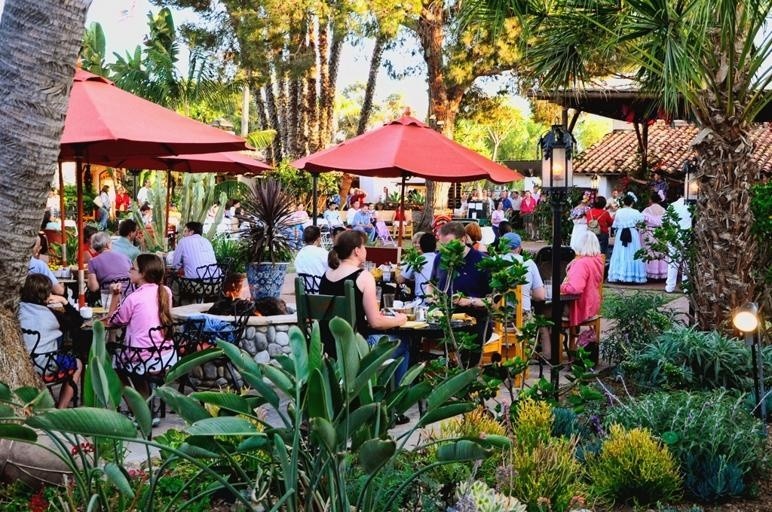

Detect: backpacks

[587,219,601,235]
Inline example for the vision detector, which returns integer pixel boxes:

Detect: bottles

[395,263,402,281]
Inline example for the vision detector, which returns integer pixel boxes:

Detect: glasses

[130,263,139,272]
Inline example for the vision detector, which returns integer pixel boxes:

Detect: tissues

[391,299,422,321]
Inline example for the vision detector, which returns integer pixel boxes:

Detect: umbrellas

[58,54,256,315]
[68,150,277,252]
[289,104,525,267]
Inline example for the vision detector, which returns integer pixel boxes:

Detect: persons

[31,169,706,426]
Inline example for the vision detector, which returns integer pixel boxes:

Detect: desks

[75,315,122,361]
[55,279,90,300]
[163,268,178,288]
[530,296,579,306]
[365,318,476,383]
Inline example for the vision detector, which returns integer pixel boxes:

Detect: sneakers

[395,414,410,425]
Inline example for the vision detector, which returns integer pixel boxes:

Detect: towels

[400,321,429,331]
[451,312,477,325]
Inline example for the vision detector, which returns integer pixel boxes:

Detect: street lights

[537,123,578,399]
[680,160,706,328]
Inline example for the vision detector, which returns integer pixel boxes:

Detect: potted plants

[241,243,292,303]
[217,238,251,296]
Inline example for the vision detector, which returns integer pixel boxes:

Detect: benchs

[340,209,413,239]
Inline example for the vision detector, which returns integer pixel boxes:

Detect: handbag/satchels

[93,195,105,208]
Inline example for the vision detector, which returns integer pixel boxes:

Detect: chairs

[101,277,139,300]
[178,263,231,305]
[500,284,530,388]
[19,328,78,409]
[295,276,356,344]
[534,247,574,291]
[106,319,205,419]
[365,247,401,268]
[222,256,233,269]
[559,254,605,366]
[42,229,76,262]
[82,209,95,223]
[187,297,255,353]
[423,292,502,378]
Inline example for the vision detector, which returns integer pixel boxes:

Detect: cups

[101,291,113,313]
[544,280,552,299]
[367,263,376,273]
[383,294,395,312]
[80,303,93,319]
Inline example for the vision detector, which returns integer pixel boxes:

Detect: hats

[501,232,522,250]
